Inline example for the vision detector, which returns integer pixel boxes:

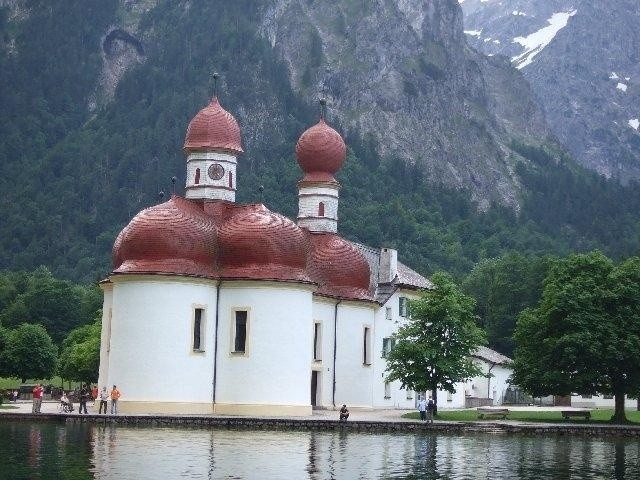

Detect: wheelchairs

[56,398,73,414]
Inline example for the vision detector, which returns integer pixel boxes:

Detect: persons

[31,384,41,413]
[426,394,437,424]
[36,382,44,413]
[99,387,109,414]
[417,395,427,422]
[78,384,90,414]
[12,390,18,403]
[60,393,75,413]
[91,385,98,405]
[339,403,349,421]
[108,384,122,415]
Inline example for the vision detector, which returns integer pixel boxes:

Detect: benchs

[562,411,591,423]
[477,409,510,420]
